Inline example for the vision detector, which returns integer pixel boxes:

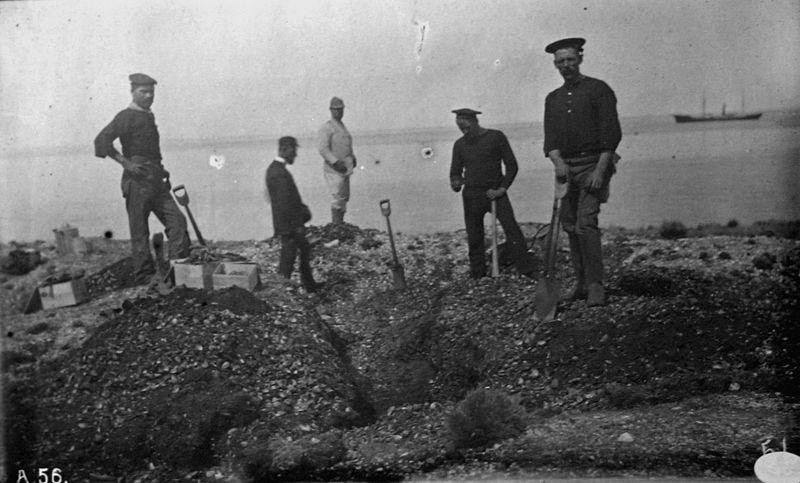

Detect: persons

[317,95,360,221]
[446,107,528,274]
[544,37,622,305]
[90,72,190,285]
[266,134,323,294]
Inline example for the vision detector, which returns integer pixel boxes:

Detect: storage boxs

[212,263,258,291]
[39,277,91,311]
[172,258,217,288]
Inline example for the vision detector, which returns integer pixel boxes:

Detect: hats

[279,136,299,148]
[129,74,157,86]
[451,109,481,120]
[545,38,585,52]
[330,97,344,110]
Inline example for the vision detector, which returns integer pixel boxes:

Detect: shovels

[380,200,405,290]
[535,174,569,320]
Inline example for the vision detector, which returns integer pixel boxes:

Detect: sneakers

[565,277,605,307]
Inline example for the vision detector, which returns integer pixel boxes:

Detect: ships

[672,86,763,123]
